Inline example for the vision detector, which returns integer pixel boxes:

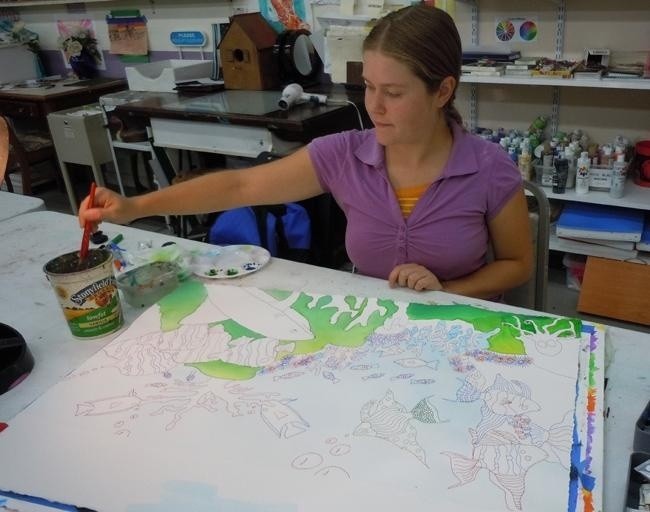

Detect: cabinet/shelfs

[410,1,650,271]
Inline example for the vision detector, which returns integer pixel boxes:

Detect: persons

[77,7,536,298]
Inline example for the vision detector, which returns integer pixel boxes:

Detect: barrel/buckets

[41,248,123,337]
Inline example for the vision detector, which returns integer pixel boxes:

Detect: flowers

[63,32,91,57]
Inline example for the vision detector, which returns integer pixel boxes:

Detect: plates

[191,244,270,277]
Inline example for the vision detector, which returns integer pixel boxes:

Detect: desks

[1,189,650,512]
[0,73,358,218]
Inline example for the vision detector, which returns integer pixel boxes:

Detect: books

[461,48,650,79]
[556,203,650,262]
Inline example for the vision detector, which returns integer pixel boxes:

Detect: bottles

[479,116,631,200]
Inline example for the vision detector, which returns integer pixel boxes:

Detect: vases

[69,50,93,78]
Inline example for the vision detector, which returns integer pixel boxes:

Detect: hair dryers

[277,83,329,112]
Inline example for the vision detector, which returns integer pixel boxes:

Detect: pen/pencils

[104,234,126,270]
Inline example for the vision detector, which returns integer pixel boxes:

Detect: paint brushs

[77,183,97,269]
